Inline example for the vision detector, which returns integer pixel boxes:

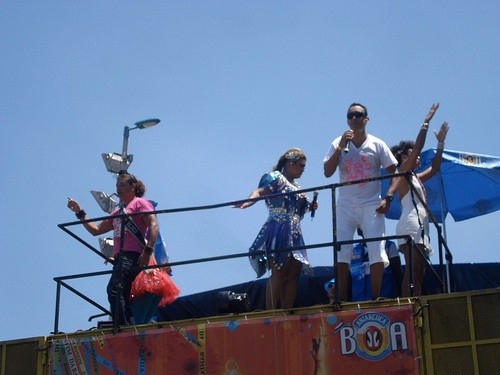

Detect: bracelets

[144,246,153,254]
[421,123,429,130]
[76,210,86,219]
[337,146,342,151]
[437,143,443,149]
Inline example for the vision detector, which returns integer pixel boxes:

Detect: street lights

[121,118,161,172]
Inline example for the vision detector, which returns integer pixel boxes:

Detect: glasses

[347,112,365,119]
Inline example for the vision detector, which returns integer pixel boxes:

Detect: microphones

[344,130,353,152]
[311,191,319,216]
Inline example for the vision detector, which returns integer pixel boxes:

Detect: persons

[324,103,400,303]
[391,102,450,297]
[67,173,159,328]
[232,148,318,310]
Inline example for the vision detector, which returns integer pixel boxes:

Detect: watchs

[386,195,392,201]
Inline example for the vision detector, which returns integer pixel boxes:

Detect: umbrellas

[380,148,500,293]
